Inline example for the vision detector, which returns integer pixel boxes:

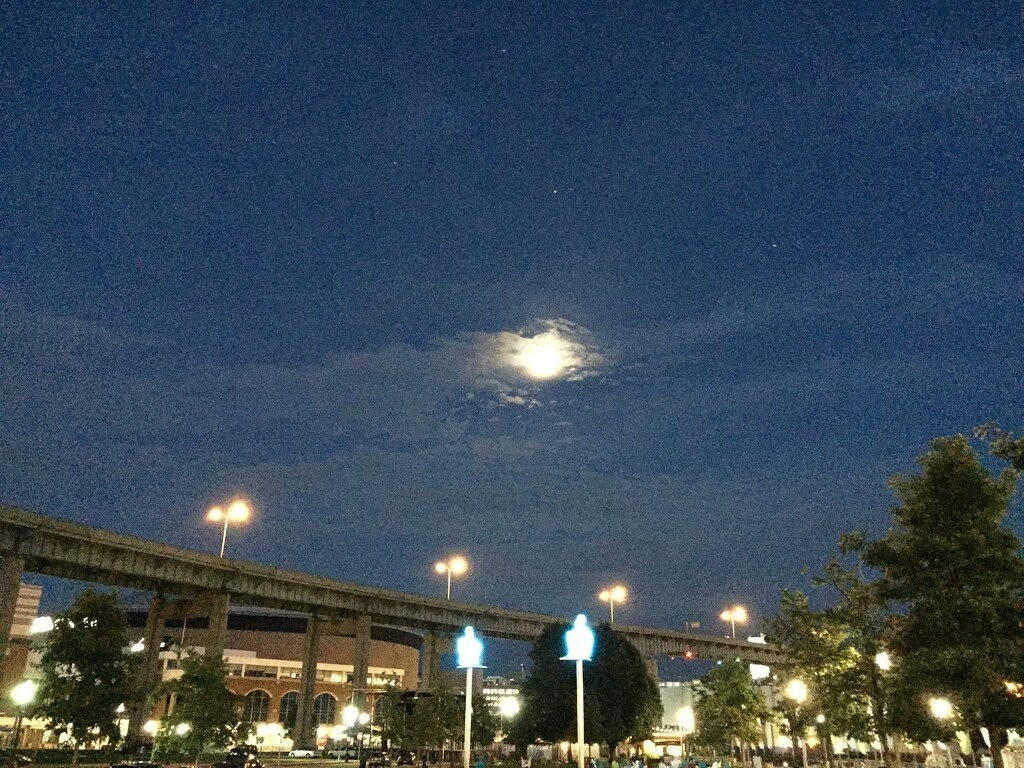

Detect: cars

[366,752,392,768]
[288,745,321,759]
[224,746,265,768]
[0,749,33,768]
[396,752,416,767]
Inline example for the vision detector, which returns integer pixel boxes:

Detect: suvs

[328,746,358,759]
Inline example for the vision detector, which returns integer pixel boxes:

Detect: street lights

[559,612,594,768]
[599,585,626,623]
[434,556,467,600]
[720,605,746,641]
[206,501,249,558]
[455,626,489,768]
